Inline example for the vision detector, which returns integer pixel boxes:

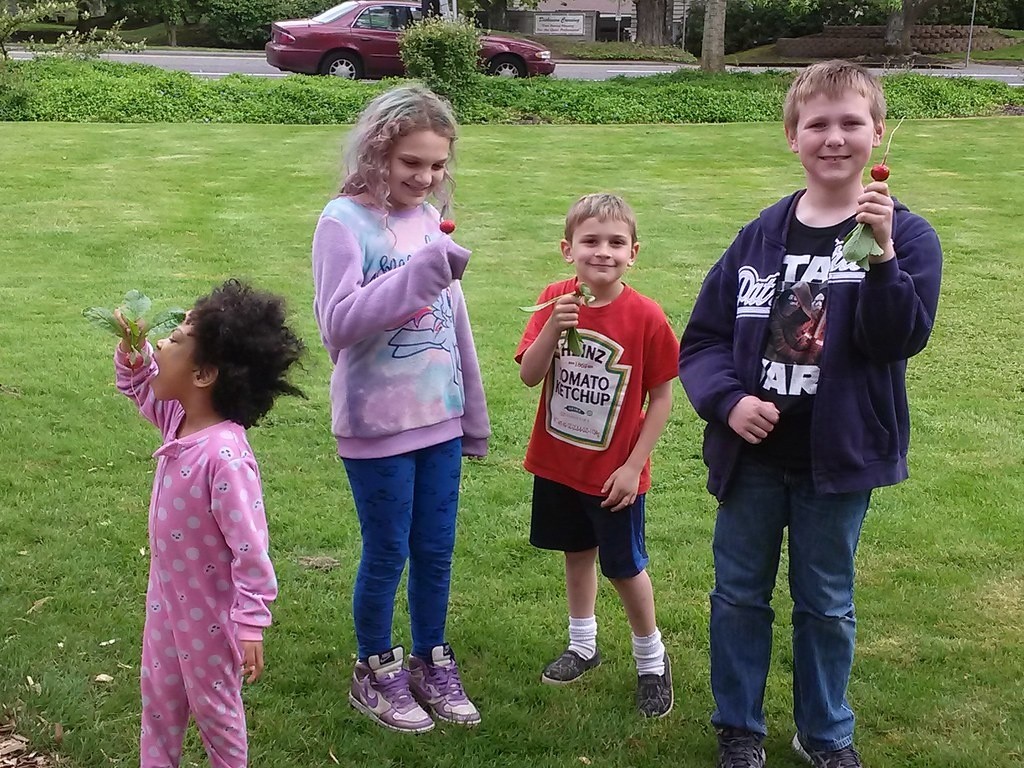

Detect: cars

[265,0,556,83]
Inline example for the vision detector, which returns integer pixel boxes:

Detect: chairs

[387,11,397,30]
[398,7,408,29]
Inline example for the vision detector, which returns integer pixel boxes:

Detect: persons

[515,194,681,720]
[677,59,944,768]
[312,86,493,735]
[111,276,311,767]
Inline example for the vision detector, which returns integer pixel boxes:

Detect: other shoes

[542,647,602,685]
[637,652,673,719]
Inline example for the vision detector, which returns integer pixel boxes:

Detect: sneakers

[408,641,481,729]
[791,731,864,768]
[717,727,767,768]
[349,645,435,734]
